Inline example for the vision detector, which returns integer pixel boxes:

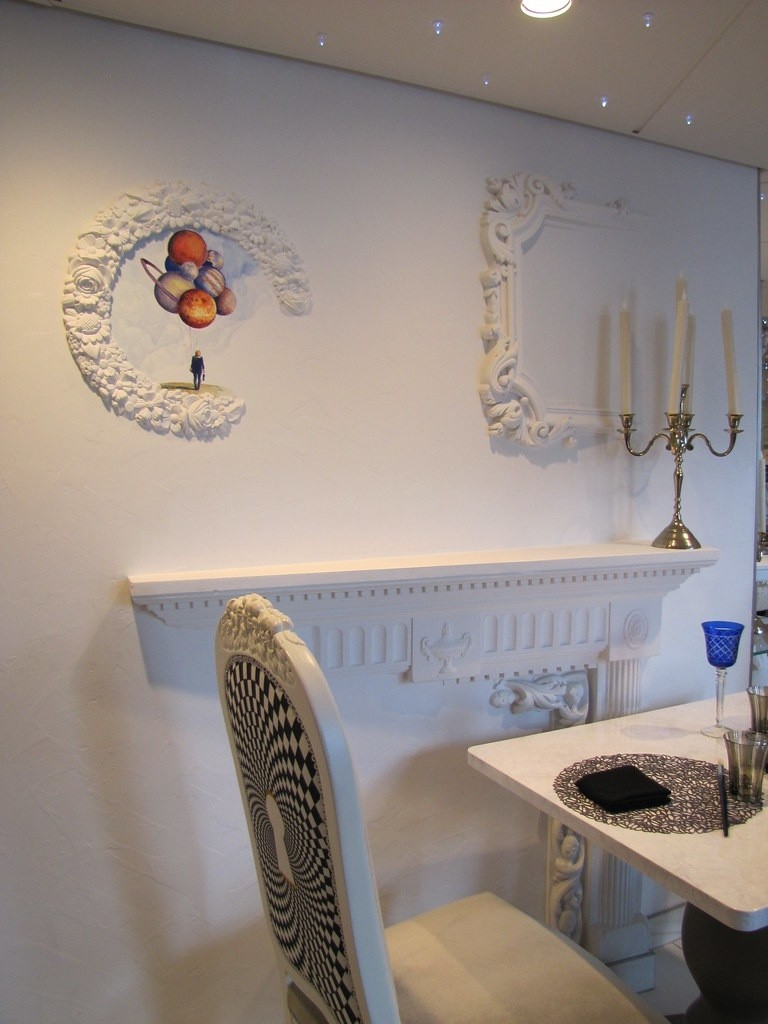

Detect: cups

[745,685,767,735]
[723,729,768,802]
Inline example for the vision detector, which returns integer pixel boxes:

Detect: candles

[685,314,693,415]
[664,290,689,414]
[614,293,639,417]
[719,307,739,417]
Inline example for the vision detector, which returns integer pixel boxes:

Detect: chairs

[211,593,646,1024]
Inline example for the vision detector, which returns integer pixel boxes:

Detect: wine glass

[699,621,744,738]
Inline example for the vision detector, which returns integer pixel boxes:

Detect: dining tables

[465,684,768,1022]
